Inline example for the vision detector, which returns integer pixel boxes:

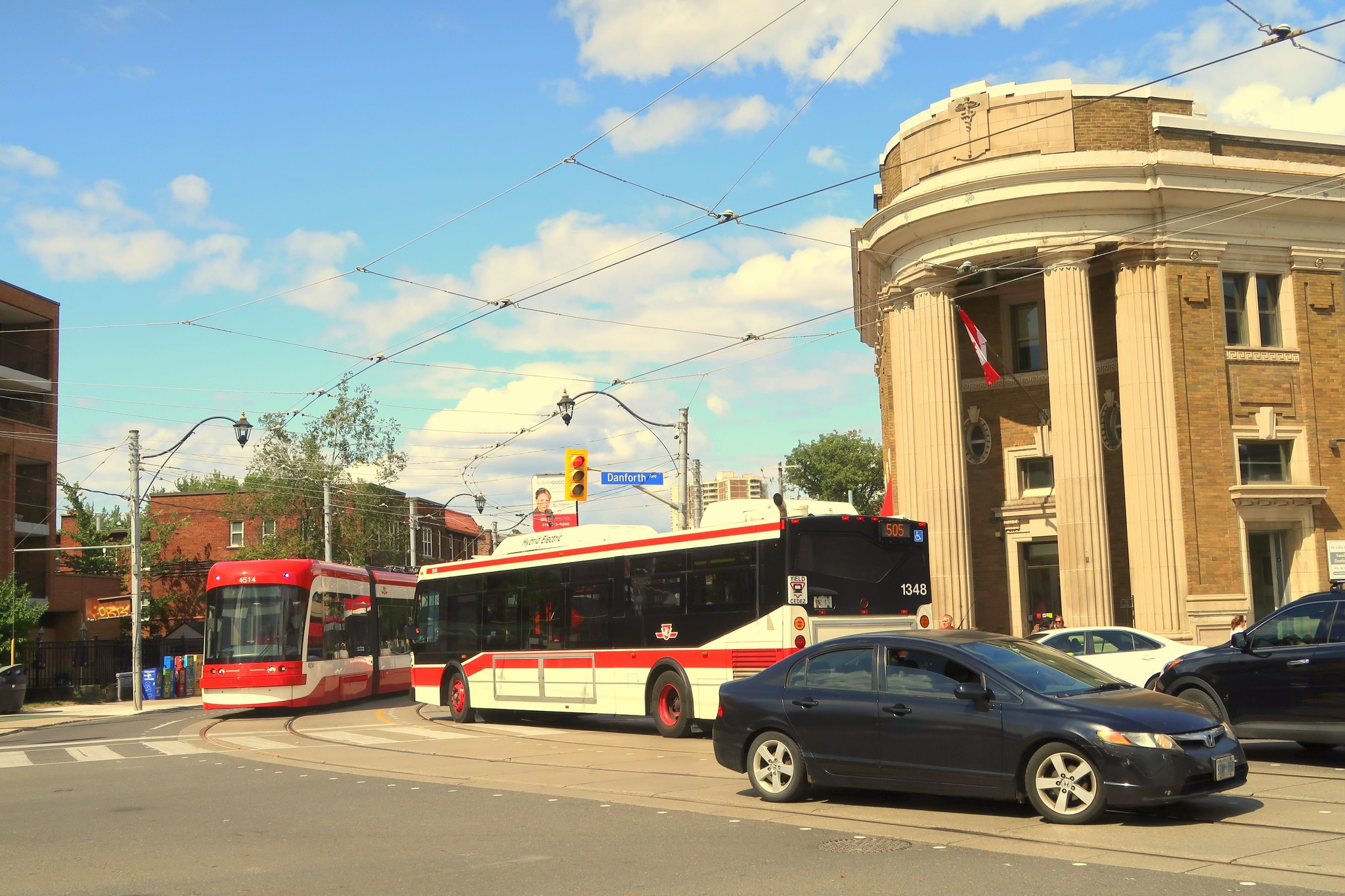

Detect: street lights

[410,493,487,567]
[125,410,254,709]
[491,511,556,553]
[558,387,690,532]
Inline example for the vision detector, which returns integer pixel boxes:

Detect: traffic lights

[564,448,589,501]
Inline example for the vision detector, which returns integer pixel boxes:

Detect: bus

[199,555,438,716]
[410,492,938,739]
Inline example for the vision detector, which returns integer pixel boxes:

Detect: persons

[1229,615,1246,642]
[533,488,553,515]
[939,614,956,630]
[1051,616,1067,629]
[1030,591,1047,623]
[1036,617,1054,633]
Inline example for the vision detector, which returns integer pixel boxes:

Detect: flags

[958,308,1001,385]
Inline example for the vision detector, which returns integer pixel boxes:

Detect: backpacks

[1030,626,1047,634]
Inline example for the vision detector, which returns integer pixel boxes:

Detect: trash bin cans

[0,664,29,713]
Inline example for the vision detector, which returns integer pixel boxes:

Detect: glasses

[1055,621,1061,624]
[1242,620,1246,623]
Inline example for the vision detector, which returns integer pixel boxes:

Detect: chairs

[1071,638,1082,651]
[899,659,920,676]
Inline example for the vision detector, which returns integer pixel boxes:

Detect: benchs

[1118,641,1149,651]
[793,659,873,686]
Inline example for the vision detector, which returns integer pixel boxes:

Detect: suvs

[1153,580,1345,752]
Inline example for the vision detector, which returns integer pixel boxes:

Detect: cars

[713,626,1250,825]
[1022,625,1209,692]
